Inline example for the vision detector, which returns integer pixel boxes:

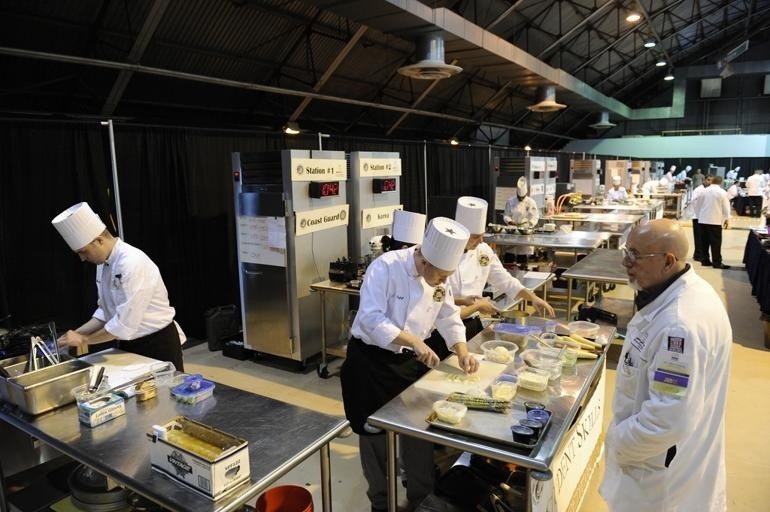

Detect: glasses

[622,248,679,263]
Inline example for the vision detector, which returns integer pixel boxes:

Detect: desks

[0,324,350,511]
[733,196,762,217]
[742,226,770,349]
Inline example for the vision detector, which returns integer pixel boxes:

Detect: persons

[691,175,715,262]
[381,209,497,319]
[448,195,556,337]
[607,166,769,218]
[49,199,184,372]
[503,176,538,270]
[699,177,731,269]
[598,218,733,512]
[341,213,480,512]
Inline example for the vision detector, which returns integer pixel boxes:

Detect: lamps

[664,74,675,81]
[626,10,640,23]
[283,122,301,135]
[644,39,656,49]
[655,59,667,67]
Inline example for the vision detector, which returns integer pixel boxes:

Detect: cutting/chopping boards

[77,348,168,390]
[413,351,507,394]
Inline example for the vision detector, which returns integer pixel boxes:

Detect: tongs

[31,336,58,370]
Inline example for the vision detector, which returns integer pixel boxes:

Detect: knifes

[399,345,466,376]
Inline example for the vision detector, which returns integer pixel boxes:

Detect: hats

[735,167,740,171]
[517,175,528,197]
[612,176,621,185]
[686,166,692,171]
[659,178,668,186]
[51,201,107,251]
[455,196,488,234]
[391,209,427,244]
[670,165,676,171]
[421,216,471,272]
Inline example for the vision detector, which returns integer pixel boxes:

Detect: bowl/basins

[487,226,503,232]
[517,229,531,234]
[505,228,516,233]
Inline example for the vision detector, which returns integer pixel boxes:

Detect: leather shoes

[713,263,730,269]
[702,263,713,266]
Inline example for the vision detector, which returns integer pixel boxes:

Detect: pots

[481,311,530,329]
